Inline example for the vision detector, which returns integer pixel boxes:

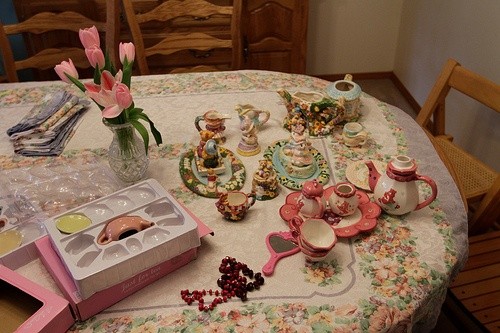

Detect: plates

[280,185,381,239]
[264,139,331,191]
[346,159,389,190]
[0,231,23,257]
[56,213,93,235]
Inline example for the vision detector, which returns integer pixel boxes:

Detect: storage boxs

[0,192,214,333]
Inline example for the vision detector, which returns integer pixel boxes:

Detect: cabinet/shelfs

[13,0,309,80]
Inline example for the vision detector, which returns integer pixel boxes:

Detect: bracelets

[181,256,265,311]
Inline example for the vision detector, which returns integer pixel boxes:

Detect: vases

[102,117,150,183]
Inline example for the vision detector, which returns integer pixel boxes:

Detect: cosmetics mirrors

[262,230,301,276]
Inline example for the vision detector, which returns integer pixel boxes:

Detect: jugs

[235,104,270,129]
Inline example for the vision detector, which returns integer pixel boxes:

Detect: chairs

[448,230,500,333]
[0,0,120,83]
[122,0,244,76]
[415,58,500,232]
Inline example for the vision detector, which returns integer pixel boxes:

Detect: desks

[0,70,469,333]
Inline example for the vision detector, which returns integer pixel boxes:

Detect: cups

[289,215,337,252]
[330,182,360,216]
[193,110,230,143]
[217,190,254,222]
[342,122,366,147]
[289,229,335,263]
[296,180,328,220]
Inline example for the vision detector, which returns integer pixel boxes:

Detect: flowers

[54,24,162,156]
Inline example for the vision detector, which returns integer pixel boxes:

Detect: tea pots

[365,155,437,216]
[276,87,345,138]
[326,72,364,121]
[98,216,155,245]
